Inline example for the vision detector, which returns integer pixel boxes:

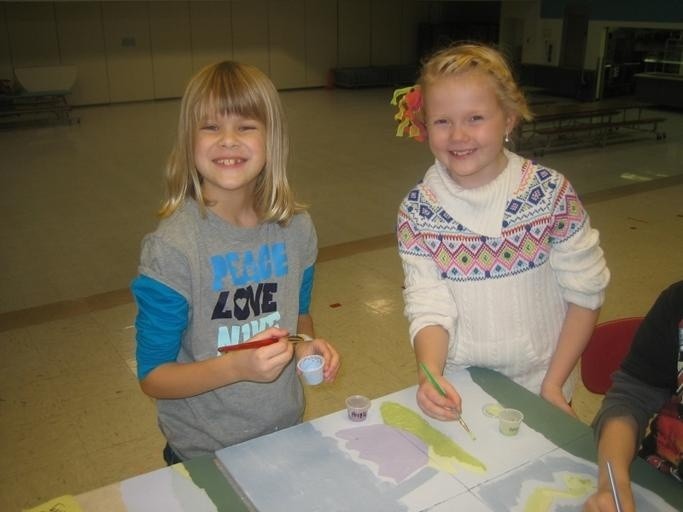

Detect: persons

[393,42,612,426]
[130,58,339,465]
[576,278,682,511]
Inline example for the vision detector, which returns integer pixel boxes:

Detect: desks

[0,89,72,103]
[516,100,656,152]
[14,367,683,512]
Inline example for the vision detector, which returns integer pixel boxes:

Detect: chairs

[581,316,646,394]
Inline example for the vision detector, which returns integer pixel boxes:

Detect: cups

[296,355,325,386]
[499,409,524,436]
[345,395,370,421]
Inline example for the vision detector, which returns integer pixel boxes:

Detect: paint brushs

[418,360,477,441]
[605,460,622,512]
[218,334,312,353]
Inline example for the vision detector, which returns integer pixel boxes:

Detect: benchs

[0,103,73,125]
[522,85,545,97]
[335,66,416,90]
[536,117,667,155]
